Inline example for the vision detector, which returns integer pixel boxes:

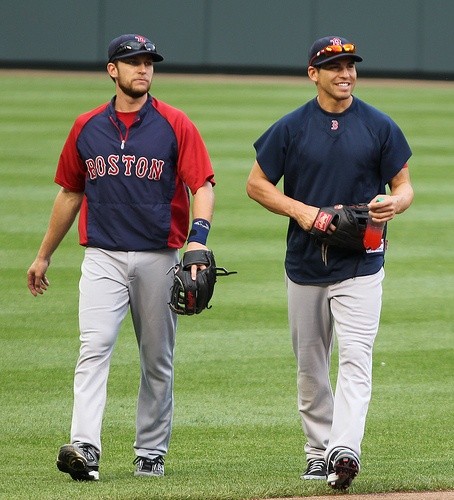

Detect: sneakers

[300,459,327,479]
[133,455,165,476]
[327,449,360,489]
[57,442,100,480]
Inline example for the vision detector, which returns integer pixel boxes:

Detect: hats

[309,35,362,66]
[108,33,163,62]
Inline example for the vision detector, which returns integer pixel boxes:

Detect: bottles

[363,197,385,250]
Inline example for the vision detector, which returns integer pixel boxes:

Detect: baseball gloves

[305,202,371,247]
[168,249,217,316]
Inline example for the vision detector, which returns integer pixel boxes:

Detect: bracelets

[187,218,211,245]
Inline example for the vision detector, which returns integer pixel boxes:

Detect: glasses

[109,41,156,59]
[309,43,357,65]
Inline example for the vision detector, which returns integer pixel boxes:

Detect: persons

[26,33,239,480]
[246,35,415,490]
